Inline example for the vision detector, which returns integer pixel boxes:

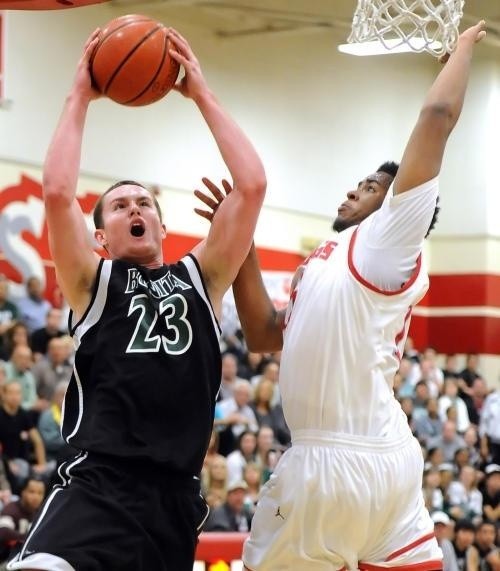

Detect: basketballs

[89,14,180,105]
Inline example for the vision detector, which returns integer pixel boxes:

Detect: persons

[0,274,500,571]
[6,24,267,571]
[193,20,487,571]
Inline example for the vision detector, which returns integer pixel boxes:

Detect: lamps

[338,18,445,58]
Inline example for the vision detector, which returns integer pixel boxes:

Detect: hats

[431,511,449,525]
